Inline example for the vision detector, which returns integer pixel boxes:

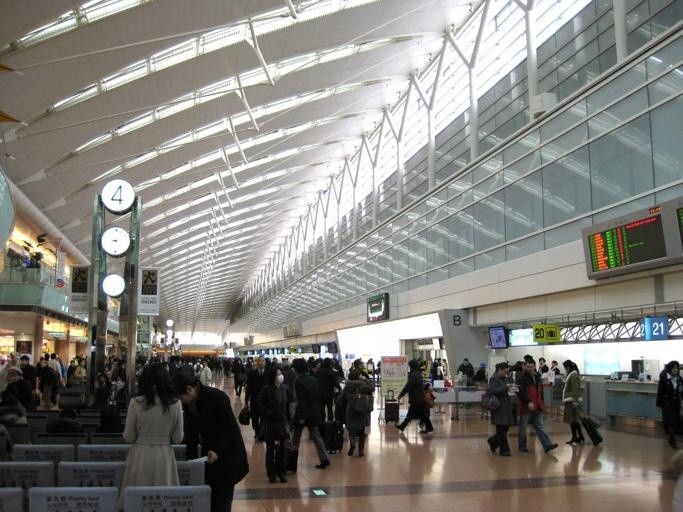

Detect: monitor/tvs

[488,326,508,349]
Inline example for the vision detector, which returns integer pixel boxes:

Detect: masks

[275,374,286,384]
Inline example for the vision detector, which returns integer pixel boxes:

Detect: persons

[172,373,248,511]
[563,360,584,444]
[246,356,373,482]
[0,353,86,409]
[121,365,184,503]
[515,360,558,453]
[487,362,513,456]
[27,256,40,277]
[457,358,474,409]
[656,361,682,449]
[477,363,487,382]
[232,356,246,398]
[538,358,549,374]
[509,361,523,383]
[94,354,225,402]
[395,357,447,433]
[550,361,559,374]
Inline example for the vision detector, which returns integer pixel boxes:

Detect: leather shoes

[315,460,331,469]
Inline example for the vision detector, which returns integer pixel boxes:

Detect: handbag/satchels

[238,402,250,425]
[480,392,501,412]
[351,396,372,414]
[588,416,601,429]
[423,388,436,408]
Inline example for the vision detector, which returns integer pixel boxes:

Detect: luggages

[274,431,298,474]
[322,420,344,454]
[573,406,604,446]
[384,390,400,425]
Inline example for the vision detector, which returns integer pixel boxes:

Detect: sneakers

[487,438,496,453]
[518,446,529,453]
[544,443,558,453]
[499,451,511,456]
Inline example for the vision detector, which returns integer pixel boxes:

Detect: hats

[7,366,23,376]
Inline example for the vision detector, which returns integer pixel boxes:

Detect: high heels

[577,436,584,444]
[566,436,578,444]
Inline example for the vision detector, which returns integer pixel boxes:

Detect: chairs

[0,409,212,511]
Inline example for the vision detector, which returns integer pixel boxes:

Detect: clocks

[98,226,131,257]
